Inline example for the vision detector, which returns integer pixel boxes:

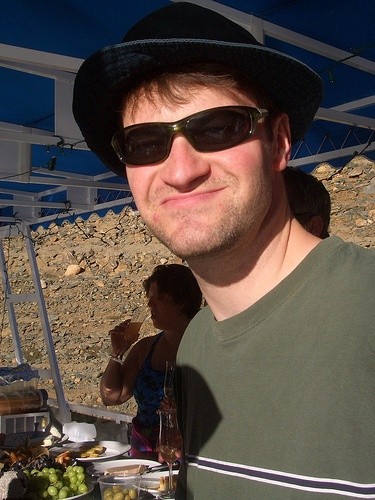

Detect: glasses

[111,105,269,167]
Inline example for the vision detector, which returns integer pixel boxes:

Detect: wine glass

[110,312,147,365]
[158,408,183,500]
[158,360,176,427]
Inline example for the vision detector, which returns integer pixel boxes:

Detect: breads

[4,446,73,464]
[158,474,178,490]
[104,464,149,476]
[4,418,62,446]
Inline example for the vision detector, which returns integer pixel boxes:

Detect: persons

[109,19,375,500]
[282,166,331,239]
[100,263,203,462]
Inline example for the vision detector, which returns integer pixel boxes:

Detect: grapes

[1,454,88,499]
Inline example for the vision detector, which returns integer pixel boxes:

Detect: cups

[97,474,142,500]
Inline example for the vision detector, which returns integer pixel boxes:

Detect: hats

[72,2,325,179]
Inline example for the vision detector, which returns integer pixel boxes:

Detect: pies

[71,445,106,458]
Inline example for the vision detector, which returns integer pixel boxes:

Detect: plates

[50,441,132,462]
[133,469,180,498]
[57,483,94,500]
[86,459,162,479]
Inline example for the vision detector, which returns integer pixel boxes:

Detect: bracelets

[110,356,122,363]
